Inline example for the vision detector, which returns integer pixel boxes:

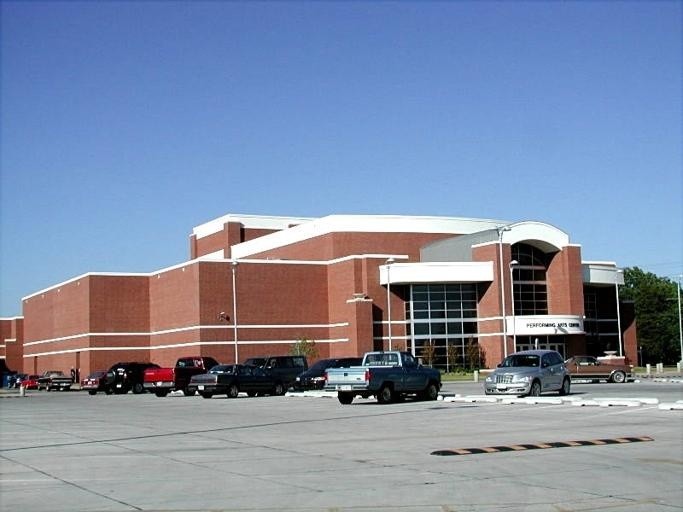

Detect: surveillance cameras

[220,311,226,317]
[582,315,586,321]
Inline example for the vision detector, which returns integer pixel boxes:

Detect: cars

[480,348,573,397]
[81,369,112,396]
[563,355,632,383]
[187,364,287,398]
[7,373,28,389]
[296,356,366,389]
[38,369,74,392]
[21,374,43,390]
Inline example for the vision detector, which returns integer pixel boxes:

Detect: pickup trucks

[143,354,221,397]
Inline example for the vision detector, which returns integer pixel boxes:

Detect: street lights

[613,269,623,356]
[669,273,682,360]
[498,224,512,362]
[384,257,396,361]
[231,259,241,365]
[507,257,520,353]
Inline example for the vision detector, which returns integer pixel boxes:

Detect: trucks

[321,348,444,406]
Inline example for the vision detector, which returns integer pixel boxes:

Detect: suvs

[98,361,164,395]
[244,355,309,393]
